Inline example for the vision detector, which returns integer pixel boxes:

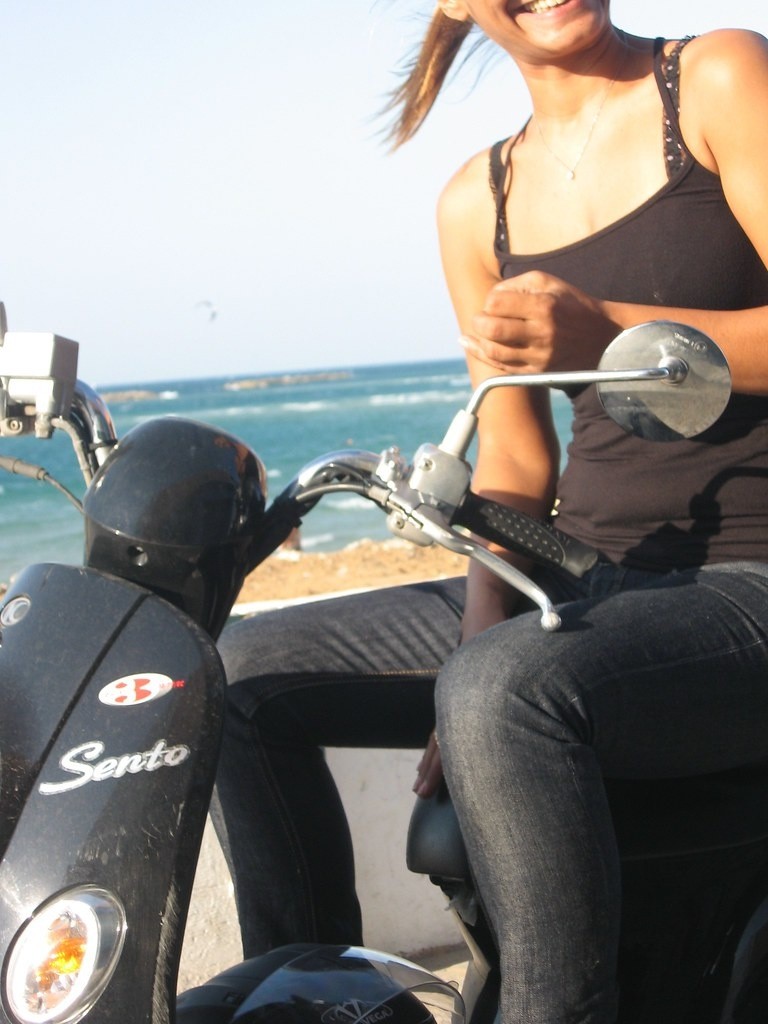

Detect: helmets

[178,942,466,1024]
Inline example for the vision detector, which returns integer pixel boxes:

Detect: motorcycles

[0,300,767,1024]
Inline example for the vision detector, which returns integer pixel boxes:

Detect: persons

[196,0,768,1024]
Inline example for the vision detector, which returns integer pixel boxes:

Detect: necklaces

[532,29,629,181]
